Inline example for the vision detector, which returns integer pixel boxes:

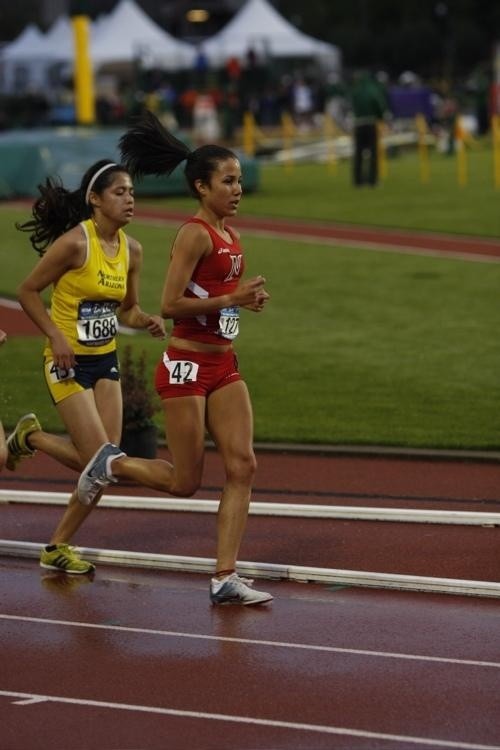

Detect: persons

[1,158,167,576]
[76,110,276,606]
[117,56,478,190]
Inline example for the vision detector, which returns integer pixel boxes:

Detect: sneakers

[76,441,128,507]
[209,571,274,607]
[39,542,96,575]
[4,413,43,472]
